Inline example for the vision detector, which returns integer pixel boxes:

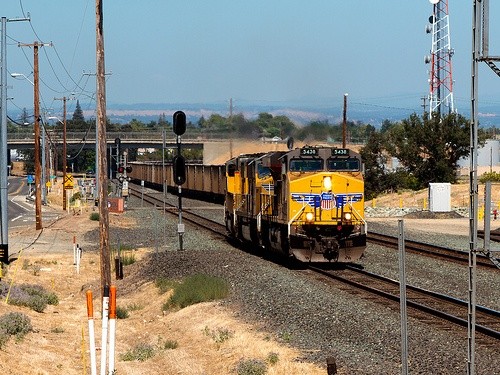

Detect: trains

[223,144,368,266]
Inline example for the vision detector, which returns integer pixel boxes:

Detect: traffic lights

[118,166,132,174]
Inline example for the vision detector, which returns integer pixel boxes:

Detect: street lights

[47,116,67,211]
[11,73,42,230]
